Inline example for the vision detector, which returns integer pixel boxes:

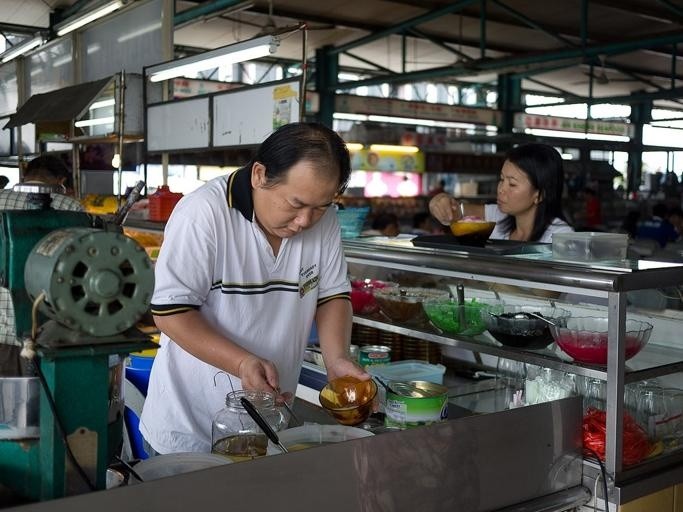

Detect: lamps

[143,26,279,87]
[46,1,126,37]
[0,31,43,65]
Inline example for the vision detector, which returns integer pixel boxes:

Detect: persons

[136,122,375,453]
[428,144,610,308]
[374,179,450,238]
[564,171,682,251]
[1,154,82,345]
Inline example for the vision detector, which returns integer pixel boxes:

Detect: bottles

[211,389,286,462]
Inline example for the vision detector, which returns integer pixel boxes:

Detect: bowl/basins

[318,374,379,426]
[550,316,653,362]
[420,297,506,336]
[482,304,570,350]
[348,279,399,315]
[128,451,235,486]
[448,221,496,248]
[265,424,375,459]
[375,287,451,326]
[338,207,370,238]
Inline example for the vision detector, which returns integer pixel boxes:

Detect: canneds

[385,380,450,431]
[358,345,393,369]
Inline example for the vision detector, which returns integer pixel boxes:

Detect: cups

[494,356,682,444]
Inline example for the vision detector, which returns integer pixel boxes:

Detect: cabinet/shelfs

[341,236,681,489]
